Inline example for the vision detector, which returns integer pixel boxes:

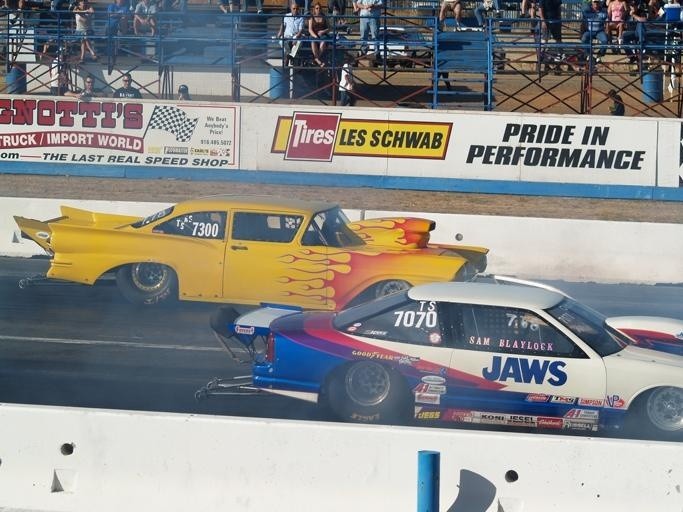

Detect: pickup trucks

[365,25,433,68]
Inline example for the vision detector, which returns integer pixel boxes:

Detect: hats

[178,84,188,93]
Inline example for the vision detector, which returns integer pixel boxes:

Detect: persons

[112,73,142,99]
[57,70,82,97]
[605,88,625,117]
[1,0,43,29]
[48,45,69,94]
[175,84,190,101]
[519,0,682,75]
[430,72,451,92]
[42,0,161,64]
[76,73,106,97]
[338,53,355,106]
[439,0,499,30]
[217,0,263,15]
[278,0,382,69]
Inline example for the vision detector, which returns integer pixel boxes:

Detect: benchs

[0,1,286,42]
[424,1,683,51]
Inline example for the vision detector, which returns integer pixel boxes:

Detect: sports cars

[13,192,490,311]
[193,282,683,440]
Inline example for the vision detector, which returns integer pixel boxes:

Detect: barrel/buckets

[642,69,664,103]
[5,63,26,93]
[269,65,289,99]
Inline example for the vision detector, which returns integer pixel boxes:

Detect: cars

[453,27,507,71]
